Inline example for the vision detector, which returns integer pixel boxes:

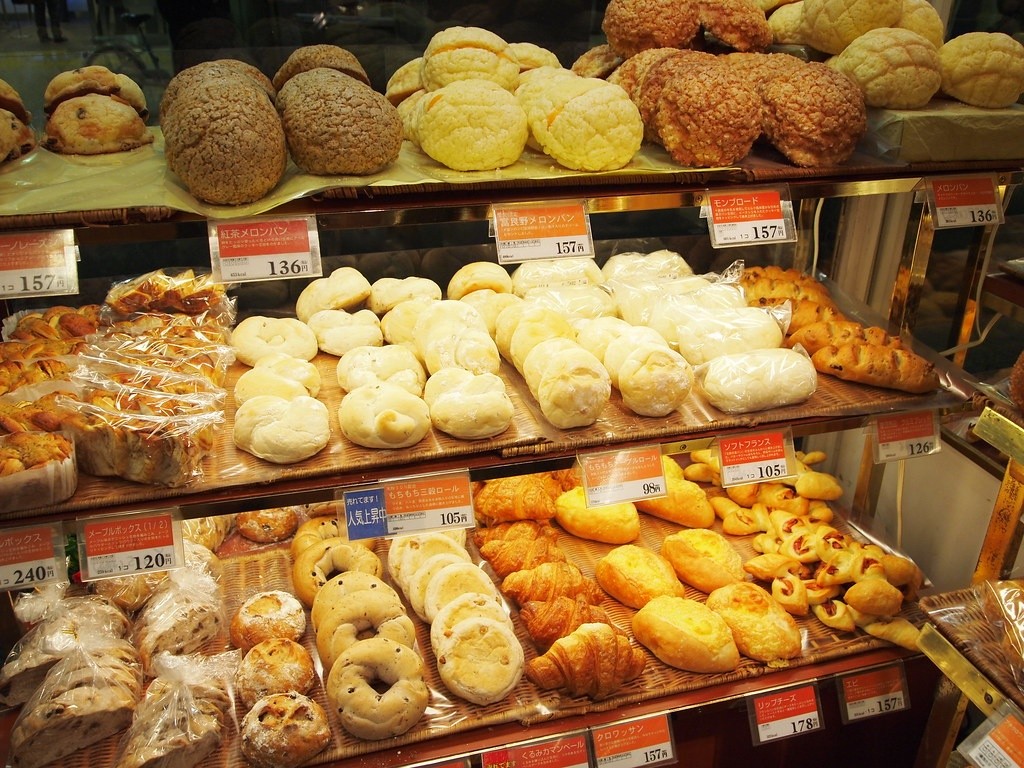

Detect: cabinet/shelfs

[0,122,1024,768]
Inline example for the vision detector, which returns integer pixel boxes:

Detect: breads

[0,0,1023,767]
